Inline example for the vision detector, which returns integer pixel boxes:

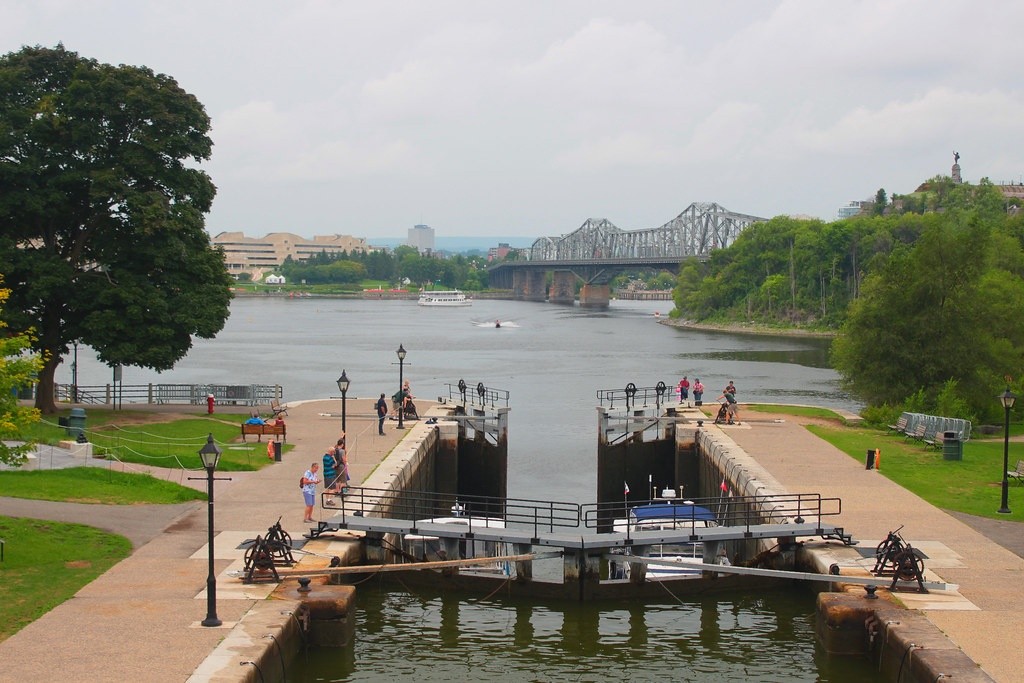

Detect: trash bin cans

[19,379,33,400]
[70,408,87,436]
[943,431,962,461]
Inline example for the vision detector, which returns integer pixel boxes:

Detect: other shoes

[326,500,335,505]
[308,518,317,522]
[304,519,312,523]
[379,433,386,435]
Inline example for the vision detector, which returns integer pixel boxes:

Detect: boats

[598,471,736,605]
[417,287,473,308]
[393,498,517,596]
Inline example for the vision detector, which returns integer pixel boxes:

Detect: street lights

[997,386,1019,513]
[394,343,407,429]
[70,361,76,384]
[197,432,224,627]
[335,368,352,449]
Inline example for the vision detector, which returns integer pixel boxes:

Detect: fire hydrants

[206,393,216,415]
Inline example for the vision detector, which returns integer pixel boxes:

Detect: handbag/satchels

[300,478,303,488]
[374,403,378,409]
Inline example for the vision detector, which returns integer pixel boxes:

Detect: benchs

[887,417,907,435]
[250,408,269,423]
[242,424,286,442]
[924,432,944,452]
[270,398,289,418]
[904,424,928,443]
[1007,460,1024,487]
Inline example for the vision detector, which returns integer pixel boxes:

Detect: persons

[391,380,416,421]
[323,431,350,505]
[378,394,388,435]
[245,414,266,425]
[680,376,690,400]
[303,463,323,522]
[693,379,704,400]
[716,389,741,425]
[953,151,960,163]
[726,381,736,397]
[273,414,285,426]
